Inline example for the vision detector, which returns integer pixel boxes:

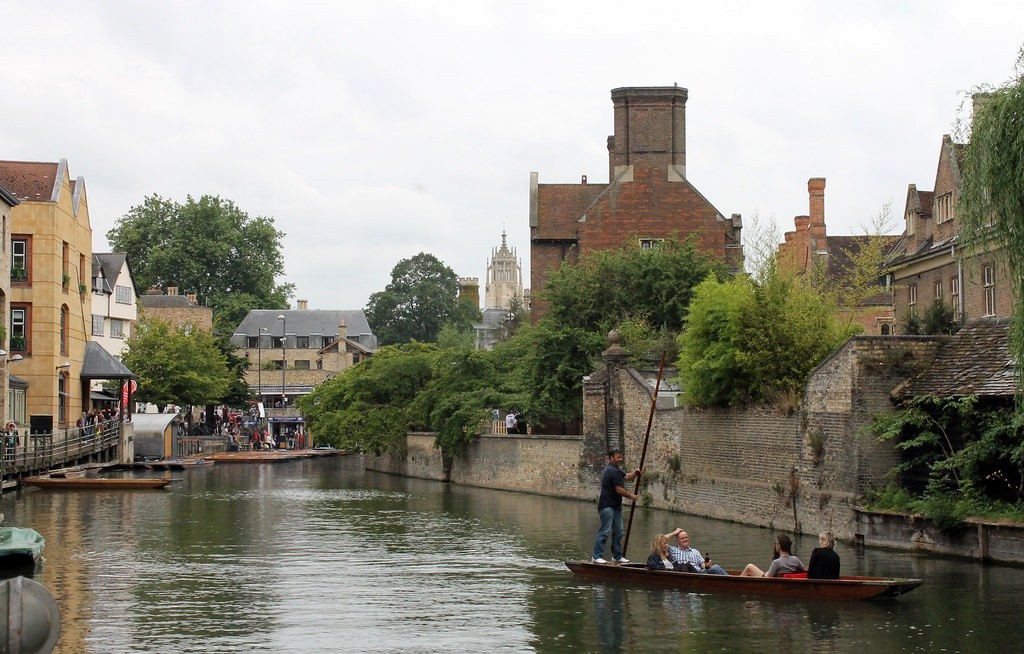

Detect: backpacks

[76,417,81,428]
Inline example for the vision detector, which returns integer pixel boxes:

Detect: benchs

[238,436,250,450]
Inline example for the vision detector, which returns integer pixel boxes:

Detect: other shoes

[82,440,87,443]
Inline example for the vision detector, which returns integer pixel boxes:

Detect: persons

[0,422,20,466]
[78,406,118,443]
[647,534,698,573]
[592,450,643,564]
[807,531,840,579]
[664,528,729,575]
[506,410,520,434]
[175,406,307,452]
[741,534,808,577]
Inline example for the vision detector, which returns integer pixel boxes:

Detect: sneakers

[612,557,629,563]
[591,556,607,563]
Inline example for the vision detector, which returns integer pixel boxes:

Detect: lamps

[56,361,71,370]
[7,354,23,364]
[0,349,6,356]
